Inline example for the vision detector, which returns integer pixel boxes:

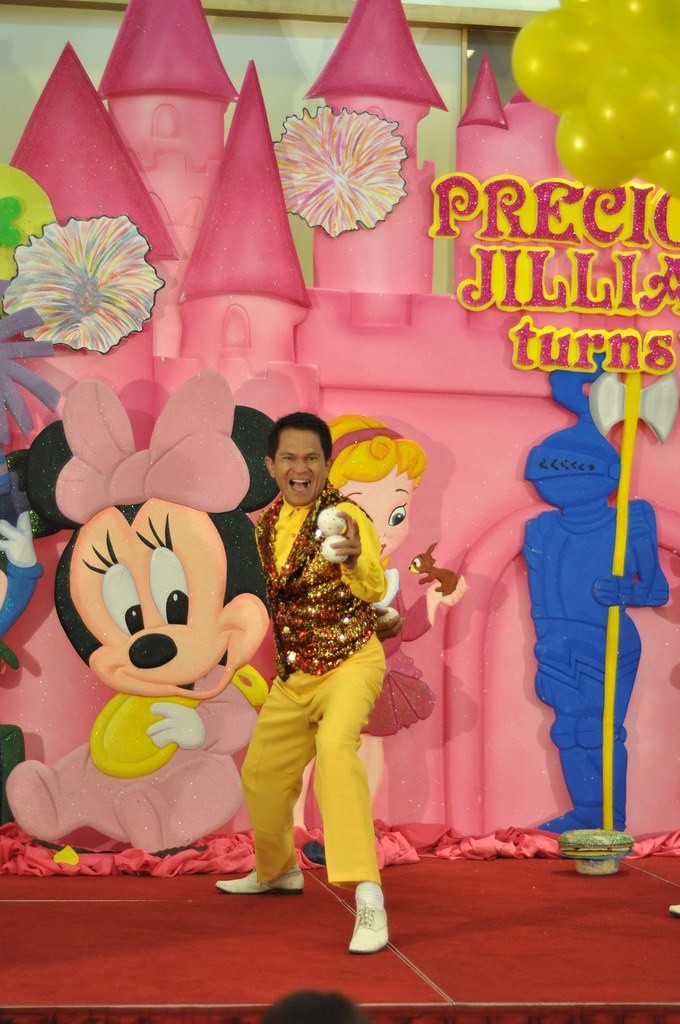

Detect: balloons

[510,0,680,200]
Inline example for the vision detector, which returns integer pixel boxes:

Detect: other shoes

[668,905,680,916]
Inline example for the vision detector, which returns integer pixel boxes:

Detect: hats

[561,852,632,875]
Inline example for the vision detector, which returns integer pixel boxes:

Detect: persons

[215,412,404,952]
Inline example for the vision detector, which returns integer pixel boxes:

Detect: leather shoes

[216,868,304,894]
[349,900,389,954]
